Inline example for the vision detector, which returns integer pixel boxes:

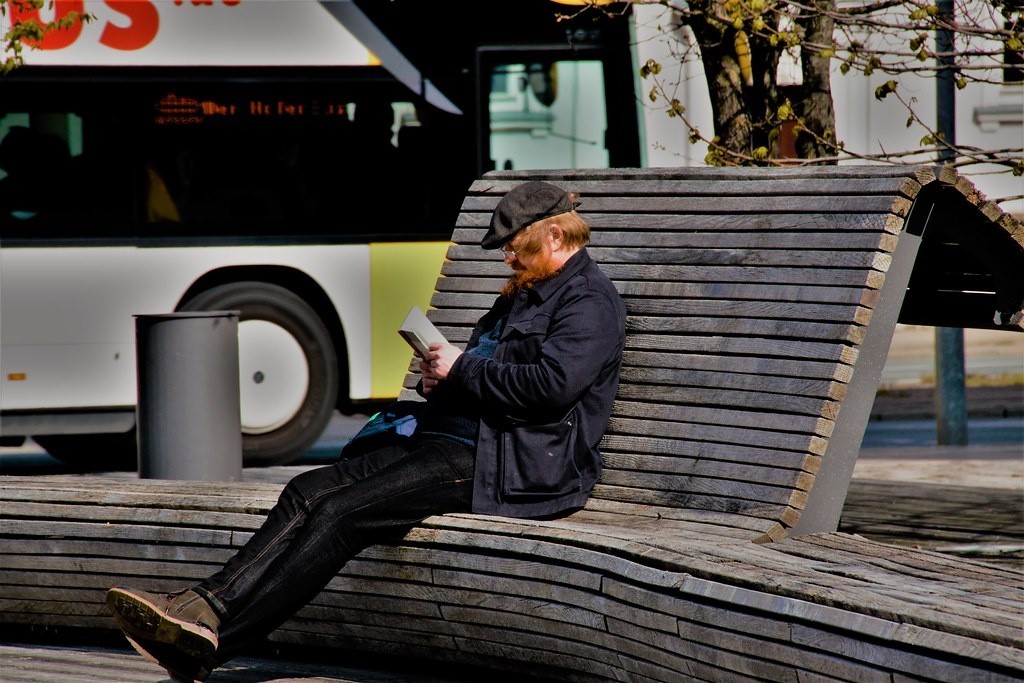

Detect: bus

[0,0,775,472]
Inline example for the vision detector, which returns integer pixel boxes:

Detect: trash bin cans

[132,309,243,485]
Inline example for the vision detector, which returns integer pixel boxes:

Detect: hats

[479,182,582,250]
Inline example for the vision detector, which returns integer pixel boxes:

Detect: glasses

[499,243,526,261]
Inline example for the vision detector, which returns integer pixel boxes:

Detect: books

[397,305,450,364]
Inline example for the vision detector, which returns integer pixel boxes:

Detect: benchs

[0,163,1024,681]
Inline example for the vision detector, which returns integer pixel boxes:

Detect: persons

[107,180,626,683]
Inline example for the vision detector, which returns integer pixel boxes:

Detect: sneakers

[119,623,216,683]
[105,585,221,658]
